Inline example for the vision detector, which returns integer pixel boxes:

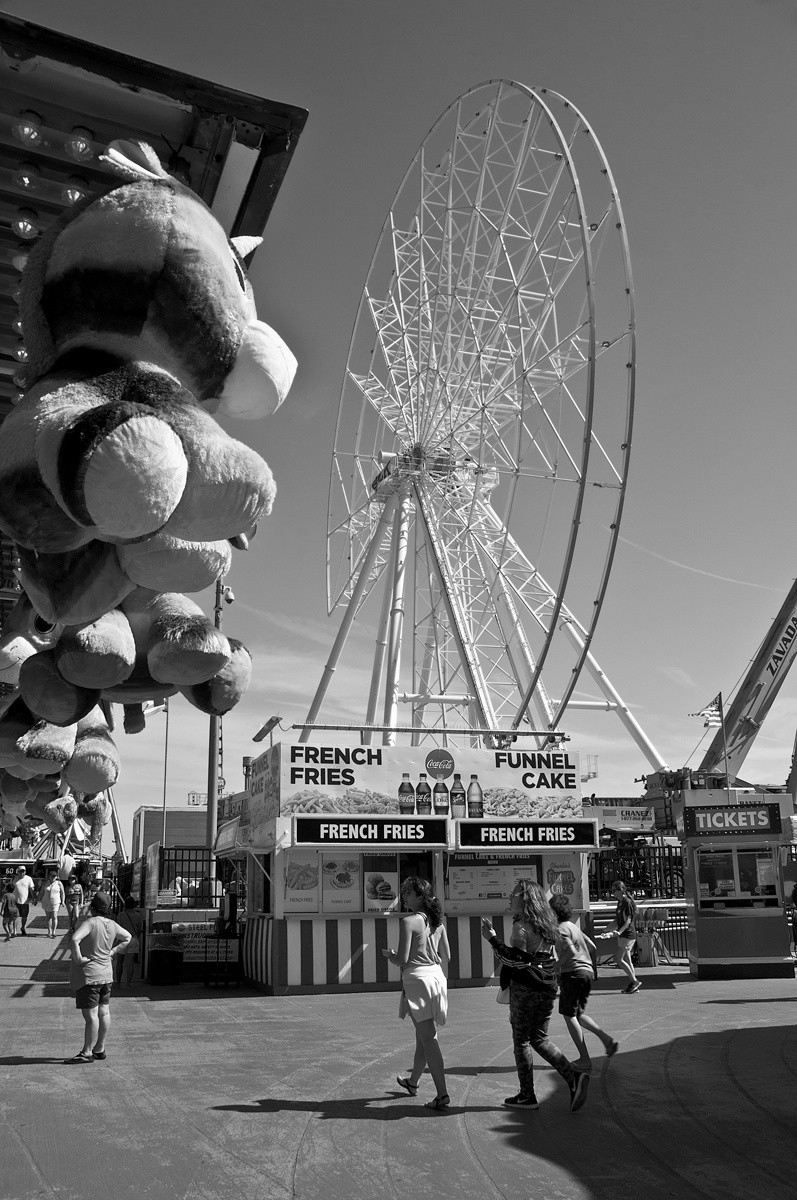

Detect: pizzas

[479,787,584,817]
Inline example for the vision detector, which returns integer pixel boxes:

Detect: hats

[71,875,76,878]
[50,872,57,876]
[18,866,26,871]
[91,892,111,909]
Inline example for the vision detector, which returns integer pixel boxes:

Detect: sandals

[425,1095,450,1111]
[621,980,642,993]
[397,1078,419,1096]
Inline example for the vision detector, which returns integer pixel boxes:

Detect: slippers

[606,1042,618,1057]
[571,1061,592,1070]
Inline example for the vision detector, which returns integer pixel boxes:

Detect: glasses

[611,889,619,892]
[68,877,75,880]
[405,890,418,898]
[510,891,524,897]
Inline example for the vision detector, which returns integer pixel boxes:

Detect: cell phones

[486,918,492,930]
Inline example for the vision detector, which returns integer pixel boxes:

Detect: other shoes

[21,928,26,936]
[47,935,56,938]
[4,933,15,941]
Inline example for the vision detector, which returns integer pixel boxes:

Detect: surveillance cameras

[225,591,234,604]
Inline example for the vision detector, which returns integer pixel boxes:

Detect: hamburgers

[366,873,396,901]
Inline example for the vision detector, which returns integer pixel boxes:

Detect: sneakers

[505,1090,539,1109]
[567,1072,589,1112]
[64,1052,94,1064]
[92,1050,106,1059]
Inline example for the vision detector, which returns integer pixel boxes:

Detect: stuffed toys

[0,139,303,864]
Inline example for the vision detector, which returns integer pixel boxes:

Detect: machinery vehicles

[597,575,796,899]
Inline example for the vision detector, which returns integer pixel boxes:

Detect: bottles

[415,773,431,813]
[601,931,615,940]
[467,774,484,818]
[433,773,449,814]
[450,773,466,818]
[398,772,415,813]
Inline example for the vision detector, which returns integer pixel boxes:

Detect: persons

[64,892,132,1063]
[549,894,618,1068]
[482,878,589,1112]
[382,876,451,1109]
[66,875,83,933]
[0,884,19,942]
[604,882,642,993]
[35,872,64,938]
[625,841,647,897]
[58,848,96,904]
[14,866,35,934]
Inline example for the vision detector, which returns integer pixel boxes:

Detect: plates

[323,863,340,874]
[330,873,358,891]
[343,861,359,872]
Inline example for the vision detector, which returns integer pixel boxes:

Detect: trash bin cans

[147,932,186,986]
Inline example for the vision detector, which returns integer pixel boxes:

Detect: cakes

[324,861,358,889]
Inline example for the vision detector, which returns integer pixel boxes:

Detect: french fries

[286,863,319,890]
[279,786,400,815]
